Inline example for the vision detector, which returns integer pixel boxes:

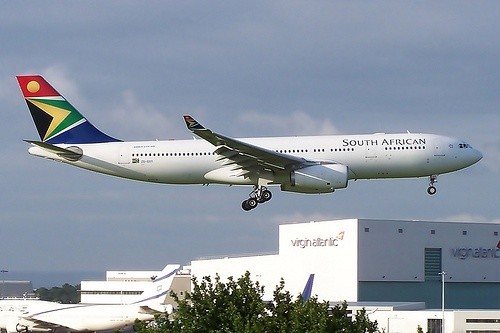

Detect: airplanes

[0,263,315,333]
[14,73,484,212]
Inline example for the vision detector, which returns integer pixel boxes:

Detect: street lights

[438,271,448,333]
